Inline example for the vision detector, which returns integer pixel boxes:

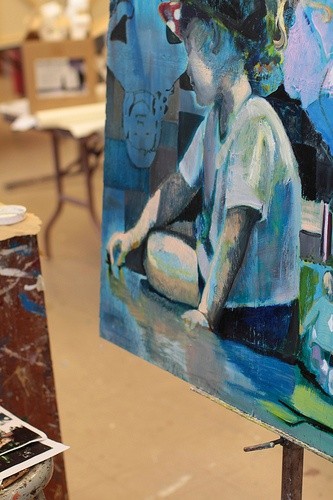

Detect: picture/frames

[20,39,101,114]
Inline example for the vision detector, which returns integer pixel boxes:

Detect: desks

[0,85,107,260]
